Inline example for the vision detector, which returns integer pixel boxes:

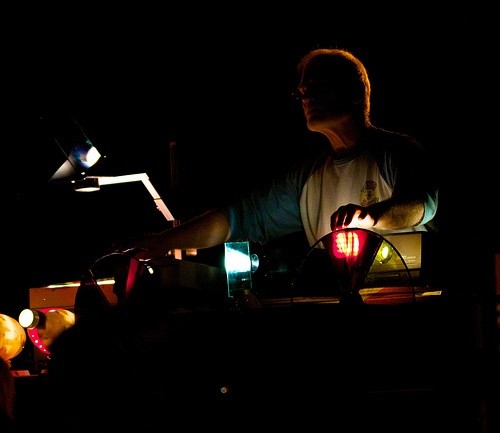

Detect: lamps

[40,115,177,225]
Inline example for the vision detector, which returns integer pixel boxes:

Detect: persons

[116,48,439,275]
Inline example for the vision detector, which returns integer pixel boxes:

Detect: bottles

[19,307,75,340]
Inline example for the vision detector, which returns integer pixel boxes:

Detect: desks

[161,284,491,432]
